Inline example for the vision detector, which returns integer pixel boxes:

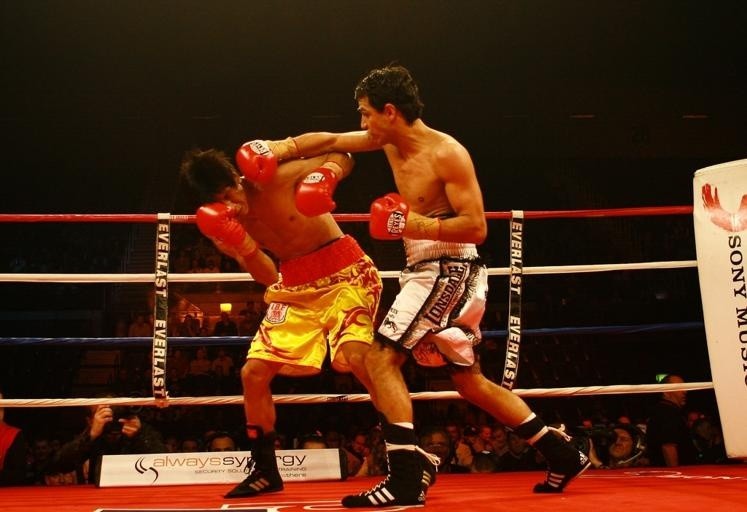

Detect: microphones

[618,451,643,464]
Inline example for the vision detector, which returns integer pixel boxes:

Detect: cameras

[109,392,142,431]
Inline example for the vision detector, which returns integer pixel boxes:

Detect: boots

[223,424,283,499]
[503,415,592,493]
[342,412,441,507]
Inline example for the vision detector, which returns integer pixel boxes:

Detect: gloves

[235,136,302,190]
[196,201,259,261]
[295,160,345,217]
[369,193,440,240]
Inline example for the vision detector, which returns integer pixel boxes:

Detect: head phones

[615,423,646,454]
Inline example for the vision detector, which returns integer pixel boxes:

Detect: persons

[2,246,740,487]
[234,65,591,494]
[178,150,440,512]
